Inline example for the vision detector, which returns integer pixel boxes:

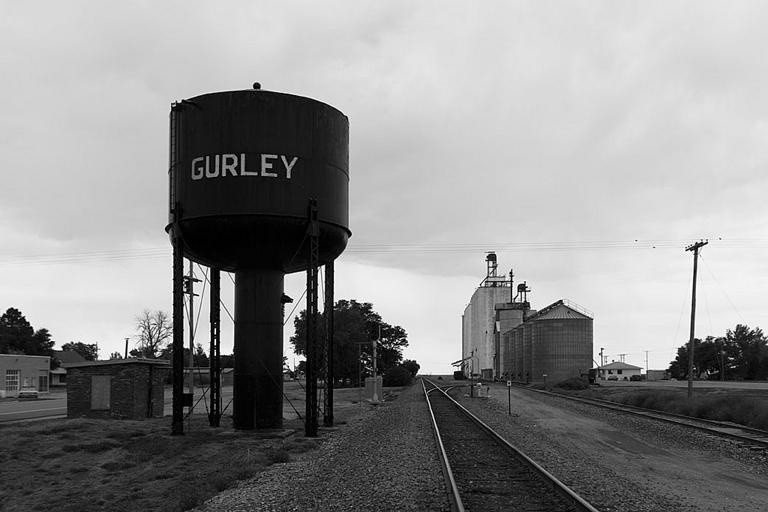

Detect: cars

[19,385,39,399]
